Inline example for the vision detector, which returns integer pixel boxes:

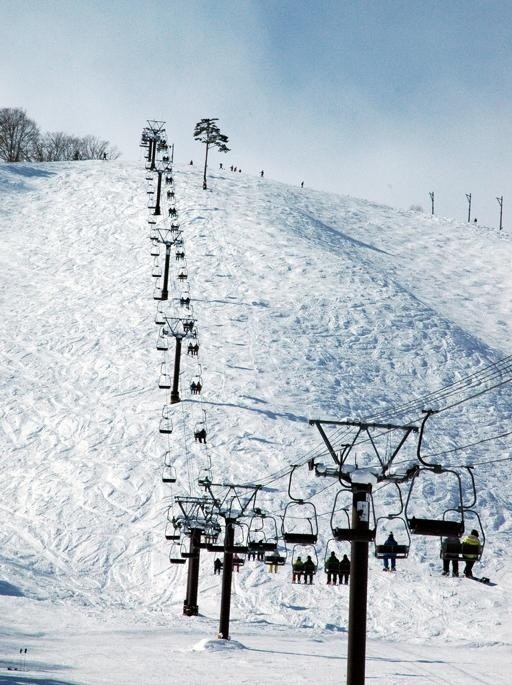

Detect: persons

[248,539,264,561]
[203,476,212,492]
[383,531,402,572]
[191,381,202,395]
[103,152,108,160]
[157,137,200,356]
[293,556,314,584]
[326,551,350,585]
[268,548,279,573]
[232,553,240,572]
[199,428,207,443]
[441,529,483,576]
[214,558,221,575]
[220,163,304,189]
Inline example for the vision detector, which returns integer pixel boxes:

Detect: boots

[384,567,396,571]
[442,570,473,577]
[293,581,348,585]
